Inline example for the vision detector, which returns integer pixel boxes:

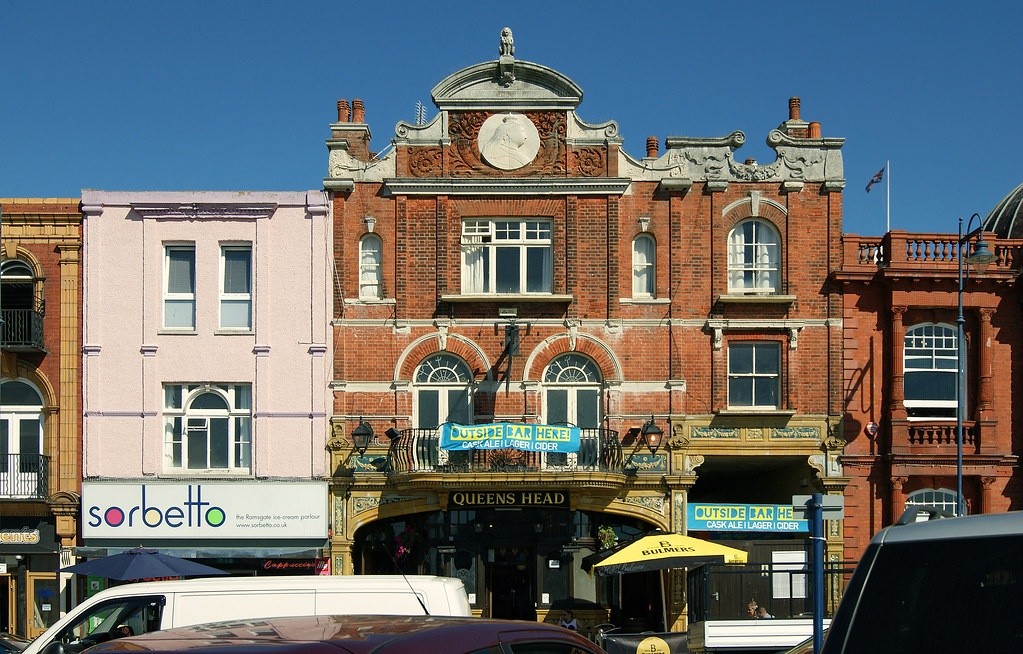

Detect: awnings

[71,546,322,559]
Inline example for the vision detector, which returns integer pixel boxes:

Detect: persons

[121,627,131,637]
[558,609,594,632]
[481,120,531,170]
[746,602,773,619]
[499,27,515,55]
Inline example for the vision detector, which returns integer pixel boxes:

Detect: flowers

[390,527,426,559]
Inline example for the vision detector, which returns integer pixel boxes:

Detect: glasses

[561,613,568,616]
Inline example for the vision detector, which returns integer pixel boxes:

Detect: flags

[865,167,885,193]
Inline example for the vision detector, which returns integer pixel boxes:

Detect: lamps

[350,415,373,459]
[643,414,664,458]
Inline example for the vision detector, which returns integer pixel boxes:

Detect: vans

[19,575,474,654]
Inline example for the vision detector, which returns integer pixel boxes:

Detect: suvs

[820,504,1023,654]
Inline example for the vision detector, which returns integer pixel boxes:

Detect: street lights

[955,213,1000,519]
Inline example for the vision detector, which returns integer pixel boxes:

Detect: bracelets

[558,619,563,623]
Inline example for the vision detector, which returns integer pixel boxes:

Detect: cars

[75,612,609,654]
[0,631,33,654]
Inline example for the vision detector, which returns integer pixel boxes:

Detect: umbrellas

[54,544,231,581]
[581,528,749,632]
